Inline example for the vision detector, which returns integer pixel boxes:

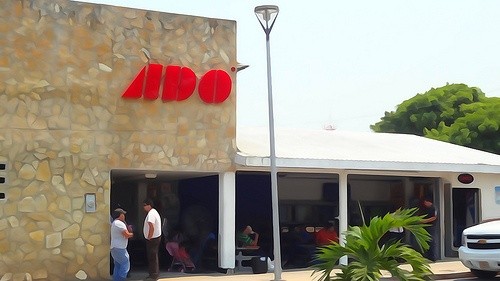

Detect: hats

[113,209,126,218]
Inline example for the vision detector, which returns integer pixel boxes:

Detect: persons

[111,209,131,281]
[420,200,438,260]
[315,223,339,244]
[240,225,288,266]
[167,232,203,269]
[143,199,162,281]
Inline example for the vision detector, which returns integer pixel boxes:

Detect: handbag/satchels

[253,261,268,274]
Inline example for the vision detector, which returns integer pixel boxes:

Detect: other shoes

[144,272,160,281]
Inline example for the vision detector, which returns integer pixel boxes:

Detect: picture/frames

[84,193,96,213]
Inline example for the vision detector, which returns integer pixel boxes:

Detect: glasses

[144,204,149,207]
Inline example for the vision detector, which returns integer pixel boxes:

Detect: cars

[457,219,500,281]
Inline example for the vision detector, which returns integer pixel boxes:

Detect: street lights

[254,4,283,281]
